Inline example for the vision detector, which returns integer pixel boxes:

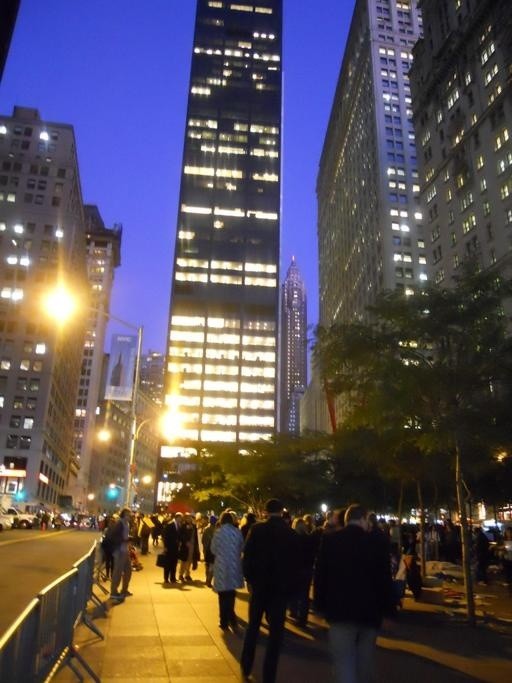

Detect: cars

[1,502,40,533]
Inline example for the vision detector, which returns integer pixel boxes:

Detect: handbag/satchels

[156,554,169,567]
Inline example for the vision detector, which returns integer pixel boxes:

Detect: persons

[200,499,392,683]
[367,514,489,581]
[69,515,81,528]
[102,508,201,599]
[90,516,96,529]
[40,512,49,528]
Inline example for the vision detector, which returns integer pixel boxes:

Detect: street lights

[40,287,167,512]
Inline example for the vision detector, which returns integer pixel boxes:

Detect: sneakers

[165,575,193,585]
[110,591,132,599]
